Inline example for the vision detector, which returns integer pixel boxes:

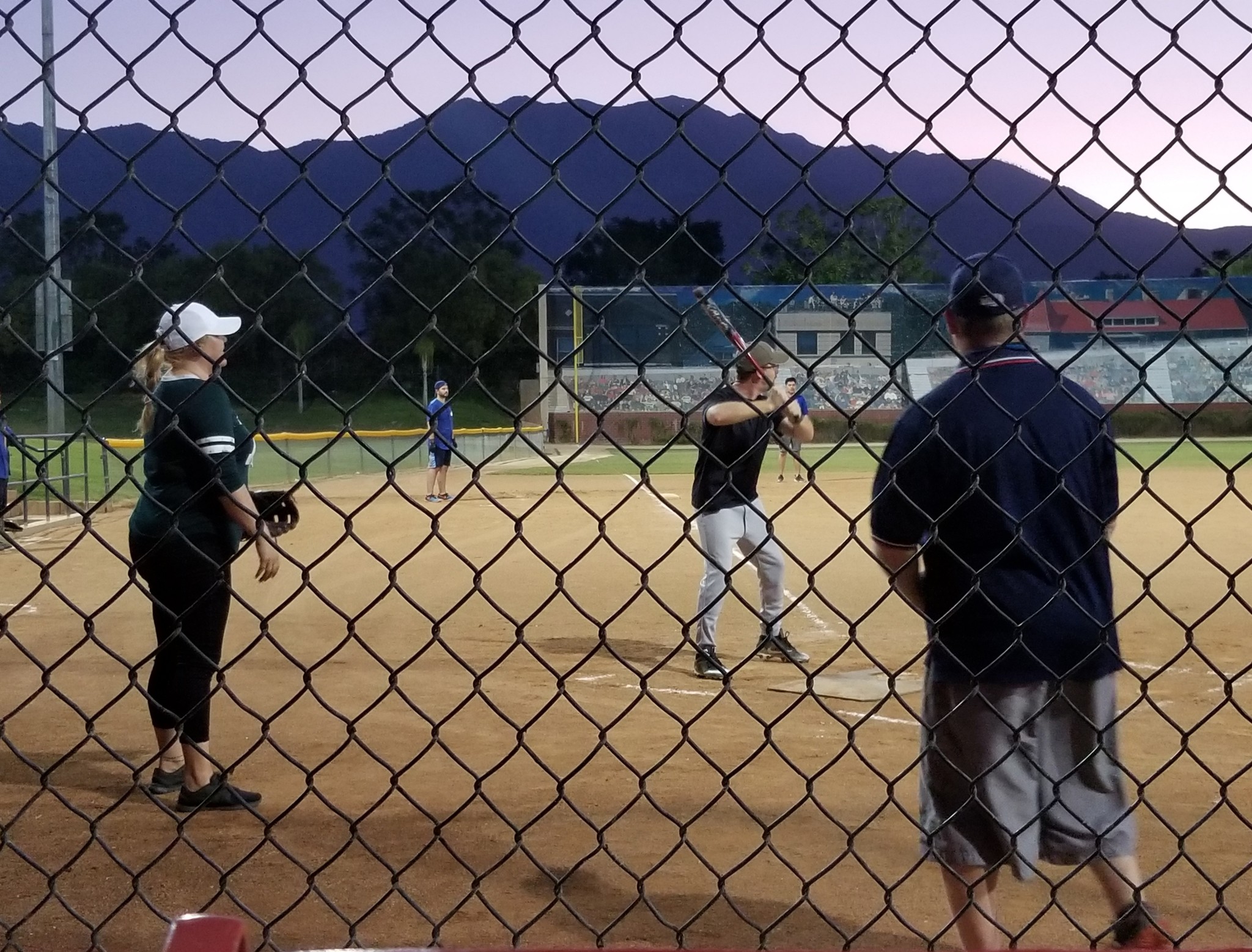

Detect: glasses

[762,362,780,373]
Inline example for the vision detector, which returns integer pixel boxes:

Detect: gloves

[429,439,436,453]
[452,439,457,448]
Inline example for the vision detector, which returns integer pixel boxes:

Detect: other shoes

[1109,908,1174,952]
[794,474,806,482]
[777,475,784,482]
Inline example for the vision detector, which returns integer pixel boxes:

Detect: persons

[426,381,454,502]
[570,346,1251,482]
[690,341,816,680]
[128,303,282,809]
[875,253,1177,952]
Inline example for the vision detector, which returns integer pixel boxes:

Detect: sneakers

[693,645,733,680]
[176,771,262,812]
[437,493,454,500]
[425,494,442,503]
[148,764,185,794]
[756,627,810,663]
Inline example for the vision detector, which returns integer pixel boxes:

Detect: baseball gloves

[240,489,301,542]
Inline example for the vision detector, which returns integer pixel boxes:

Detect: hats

[736,340,789,371]
[434,380,447,397]
[156,302,241,351]
[950,251,1026,316]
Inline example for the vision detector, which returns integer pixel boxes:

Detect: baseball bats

[694,285,800,420]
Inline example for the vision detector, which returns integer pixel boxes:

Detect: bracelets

[789,413,804,424]
